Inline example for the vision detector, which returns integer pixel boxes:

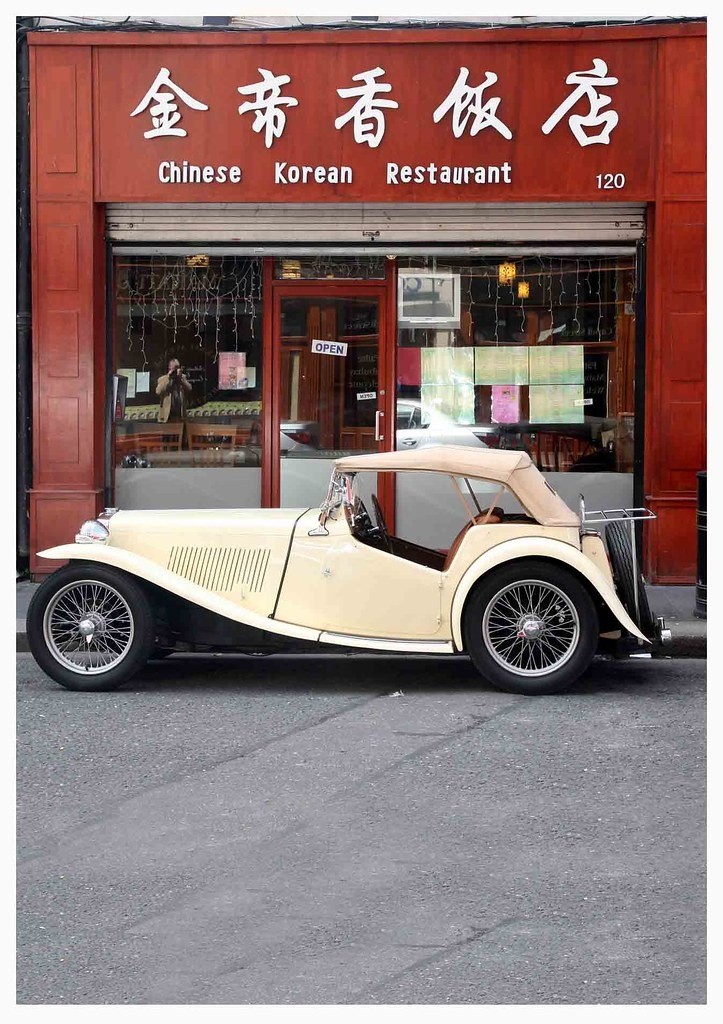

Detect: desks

[113,415,257,445]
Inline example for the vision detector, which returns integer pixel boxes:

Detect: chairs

[445,506,504,572]
[186,423,237,467]
[134,422,184,468]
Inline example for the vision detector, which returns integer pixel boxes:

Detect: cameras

[171,366,187,378]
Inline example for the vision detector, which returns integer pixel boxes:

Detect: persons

[156,358,192,451]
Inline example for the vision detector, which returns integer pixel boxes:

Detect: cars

[395,396,496,451]
[25,444,673,695]
